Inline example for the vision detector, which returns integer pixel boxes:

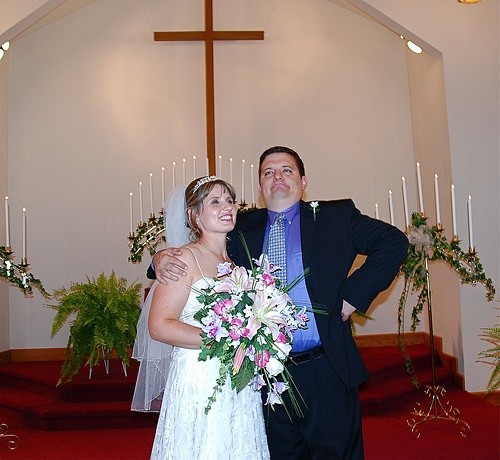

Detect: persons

[130,176,271,460]
[146,147,408,460]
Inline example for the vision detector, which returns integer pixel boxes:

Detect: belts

[285,348,321,366]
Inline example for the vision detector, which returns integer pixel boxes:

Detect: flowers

[309,200,320,223]
[192,231,331,417]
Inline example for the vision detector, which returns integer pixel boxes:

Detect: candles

[160,167,166,207]
[415,162,425,213]
[22,208,26,257]
[374,203,379,219]
[218,156,255,203]
[149,172,154,212]
[388,189,394,225]
[129,191,134,235]
[138,181,143,221]
[4,196,10,248]
[451,184,457,236]
[192,155,197,178]
[205,158,209,176]
[173,161,176,193]
[182,159,186,185]
[467,195,475,248]
[434,174,440,224]
[402,176,411,226]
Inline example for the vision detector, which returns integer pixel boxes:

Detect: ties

[267,214,289,290]
